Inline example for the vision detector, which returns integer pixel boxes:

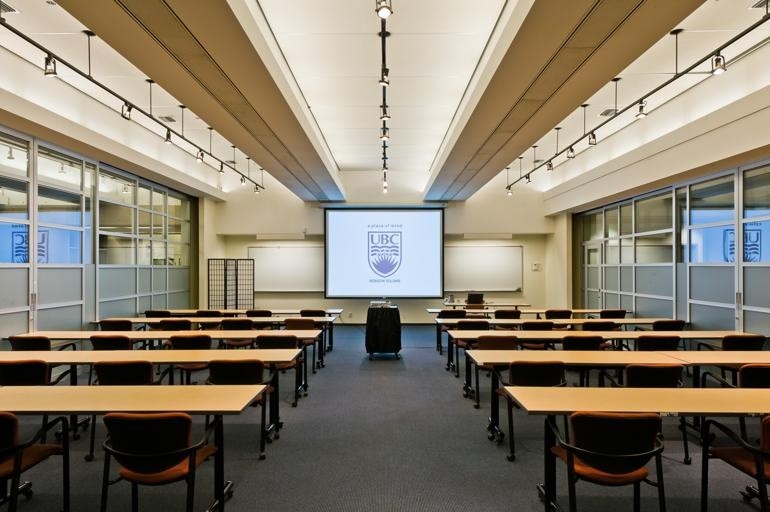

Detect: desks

[466,349,769,446]
[1,330,324,398]
[502,385,769,510]
[2,349,303,444]
[2,385,269,511]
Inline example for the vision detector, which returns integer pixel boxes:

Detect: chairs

[8,336,64,386]
[543,411,666,510]
[486,361,572,462]
[87,301,343,374]
[423,292,769,409]
[98,412,218,510]
[84,361,160,461]
[1,360,52,444]
[701,363,769,443]
[601,363,693,465]
[2,411,70,510]
[699,413,769,511]
[170,335,212,386]
[202,360,275,462]
[253,335,306,408]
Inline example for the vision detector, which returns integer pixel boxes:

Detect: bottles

[450,295,454,302]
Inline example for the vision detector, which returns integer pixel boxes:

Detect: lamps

[501,0,770,195]
[375,0,393,197]
[0,0,265,194]
